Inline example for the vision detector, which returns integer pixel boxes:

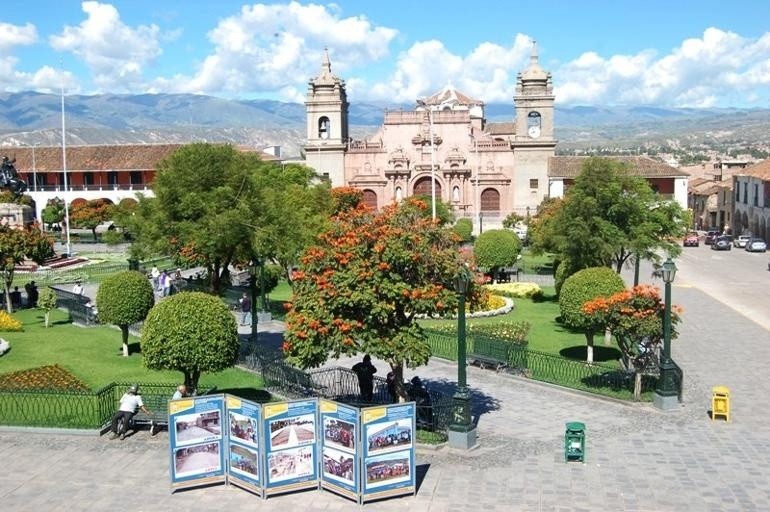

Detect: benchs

[464,336,513,374]
[111,393,175,437]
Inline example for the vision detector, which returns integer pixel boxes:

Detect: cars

[683,229,768,253]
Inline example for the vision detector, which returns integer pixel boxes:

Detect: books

[238,298,244,304]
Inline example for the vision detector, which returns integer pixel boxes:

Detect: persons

[227,416,257,475]
[384,362,397,399]
[171,385,188,400]
[238,292,253,328]
[71,280,85,298]
[322,419,411,483]
[408,376,433,418]
[11,286,21,296]
[106,382,151,442]
[26,281,39,309]
[0,155,28,199]
[140,261,183,299]
[351,353,379,402]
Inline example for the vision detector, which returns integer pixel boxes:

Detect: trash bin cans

[712,386,730,422]
[565,422,585,464]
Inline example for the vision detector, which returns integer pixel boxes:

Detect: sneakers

[109,432,116,440]
[119,433,125,440]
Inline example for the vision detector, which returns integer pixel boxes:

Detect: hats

[129,383,138,393]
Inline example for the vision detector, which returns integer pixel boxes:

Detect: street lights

[414,98,438,225]
[451,269,475,432]
[656,257,679,395]
[526,206,532,226]
[478,212,485,233]
[19,141,41,192]
